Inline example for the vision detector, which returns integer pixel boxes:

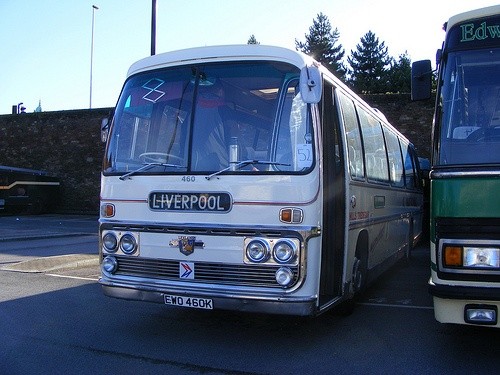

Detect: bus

[425,2,500,345]
[95,44,416,330]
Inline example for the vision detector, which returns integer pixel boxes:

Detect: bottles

[228,136,242,171]
